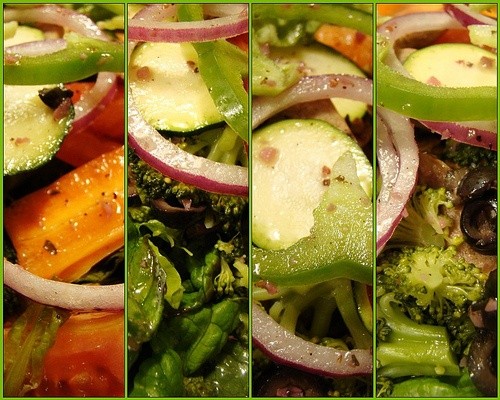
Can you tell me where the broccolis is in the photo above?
[380,247,489,326]
[391,184,453,249]
[128,124,249,258]
